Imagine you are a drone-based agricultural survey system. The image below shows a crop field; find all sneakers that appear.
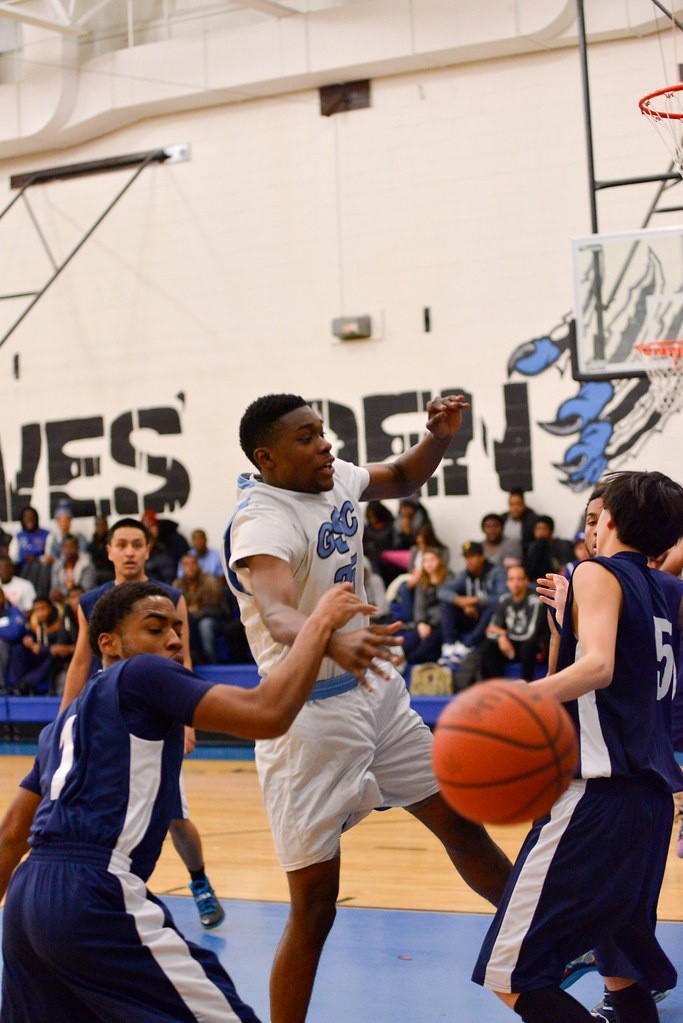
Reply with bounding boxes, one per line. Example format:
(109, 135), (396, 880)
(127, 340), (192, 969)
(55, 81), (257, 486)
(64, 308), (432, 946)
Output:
(189, 876), (225, 930)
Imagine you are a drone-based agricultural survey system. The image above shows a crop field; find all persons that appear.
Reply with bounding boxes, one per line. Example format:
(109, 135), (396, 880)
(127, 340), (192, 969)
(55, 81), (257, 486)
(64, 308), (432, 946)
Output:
(0, 393), (683, 1023)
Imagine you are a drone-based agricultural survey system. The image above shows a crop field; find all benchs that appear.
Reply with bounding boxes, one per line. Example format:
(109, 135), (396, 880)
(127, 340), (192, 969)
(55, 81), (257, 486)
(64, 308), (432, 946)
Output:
(0, 665), (547, 741)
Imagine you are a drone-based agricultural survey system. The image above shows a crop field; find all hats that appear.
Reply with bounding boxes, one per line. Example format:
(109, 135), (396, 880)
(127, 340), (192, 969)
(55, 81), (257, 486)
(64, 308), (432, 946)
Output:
(460, 542), (483, 554)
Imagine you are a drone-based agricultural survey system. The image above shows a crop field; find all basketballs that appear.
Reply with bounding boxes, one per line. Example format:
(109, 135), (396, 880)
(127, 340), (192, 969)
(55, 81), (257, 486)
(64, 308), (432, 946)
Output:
(433, 675), (580, 828)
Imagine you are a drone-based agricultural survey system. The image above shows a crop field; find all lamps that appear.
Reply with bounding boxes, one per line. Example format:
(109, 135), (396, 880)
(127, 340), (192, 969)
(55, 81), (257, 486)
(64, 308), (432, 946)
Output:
(332, 315), (372, 341)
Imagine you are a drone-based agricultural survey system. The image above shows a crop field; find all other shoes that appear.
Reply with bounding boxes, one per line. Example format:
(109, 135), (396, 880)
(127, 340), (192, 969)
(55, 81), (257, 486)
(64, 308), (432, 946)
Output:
(591, 987), (671, 1018)
(12, 681), (29, 696)
(449, 640), (474, 664)
(675, 811), (683, 858)
(559, 949), (600, 989)
(437, 643), (453, 666)
(0, 687), (12, 695)
